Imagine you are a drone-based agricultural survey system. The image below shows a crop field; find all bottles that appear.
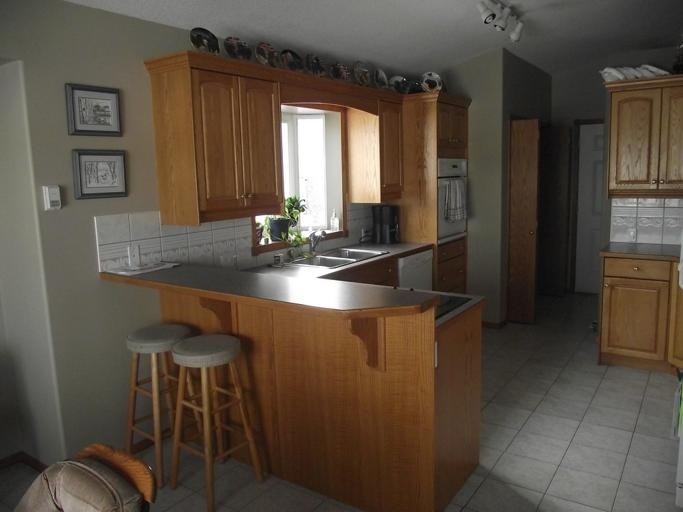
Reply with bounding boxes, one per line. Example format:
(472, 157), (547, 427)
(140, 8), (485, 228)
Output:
(273, 250), (283, 265)
(331, 209), (339, 231)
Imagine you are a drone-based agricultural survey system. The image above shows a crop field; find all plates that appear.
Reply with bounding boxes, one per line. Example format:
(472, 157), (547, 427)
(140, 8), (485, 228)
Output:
(188, 27), (443, 94)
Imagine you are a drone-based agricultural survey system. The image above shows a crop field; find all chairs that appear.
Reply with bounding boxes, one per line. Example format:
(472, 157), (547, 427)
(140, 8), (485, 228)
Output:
(71, 443), (156, 502)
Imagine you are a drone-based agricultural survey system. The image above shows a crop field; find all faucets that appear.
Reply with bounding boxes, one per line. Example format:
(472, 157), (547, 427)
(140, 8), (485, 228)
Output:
(309, 230), (326, 251)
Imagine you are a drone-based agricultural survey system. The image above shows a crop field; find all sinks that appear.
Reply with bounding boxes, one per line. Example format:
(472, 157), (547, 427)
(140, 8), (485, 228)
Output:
(316, 247), (388, 261)
(289, 255), (358, 270)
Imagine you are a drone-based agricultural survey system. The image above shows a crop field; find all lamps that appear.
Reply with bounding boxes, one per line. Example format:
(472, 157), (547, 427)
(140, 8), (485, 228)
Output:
(476, 0), (525, 42)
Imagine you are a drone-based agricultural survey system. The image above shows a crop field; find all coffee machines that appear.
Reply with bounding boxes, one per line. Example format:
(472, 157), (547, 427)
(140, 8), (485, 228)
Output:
(371, 205), (400, 245)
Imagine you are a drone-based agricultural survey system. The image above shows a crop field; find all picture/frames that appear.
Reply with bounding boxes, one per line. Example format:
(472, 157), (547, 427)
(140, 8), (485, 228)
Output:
(65, 83), (122, 137)
(72, 149), (127, 199)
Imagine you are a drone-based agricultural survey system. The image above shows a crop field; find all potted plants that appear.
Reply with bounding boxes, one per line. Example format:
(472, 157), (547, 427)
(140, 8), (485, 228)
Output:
(267, 195), (306, 247)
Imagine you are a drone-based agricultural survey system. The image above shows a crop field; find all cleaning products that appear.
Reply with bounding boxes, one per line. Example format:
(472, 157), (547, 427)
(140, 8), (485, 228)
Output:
(375, 222), (381, 243)
(272, 249), (284, 268)
(330, 208), (339, 232)
(383, 222), (390, 244)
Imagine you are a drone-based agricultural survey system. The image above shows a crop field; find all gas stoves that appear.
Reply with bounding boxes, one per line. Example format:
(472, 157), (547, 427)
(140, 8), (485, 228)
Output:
(389, 286), (472, 320)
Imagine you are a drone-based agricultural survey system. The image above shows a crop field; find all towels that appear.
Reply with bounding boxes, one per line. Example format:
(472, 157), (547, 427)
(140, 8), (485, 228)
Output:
(444, 179), (468, 224)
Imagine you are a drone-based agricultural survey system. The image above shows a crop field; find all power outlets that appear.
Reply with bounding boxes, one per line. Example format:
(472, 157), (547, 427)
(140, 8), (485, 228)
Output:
(362, 228), (368, 243)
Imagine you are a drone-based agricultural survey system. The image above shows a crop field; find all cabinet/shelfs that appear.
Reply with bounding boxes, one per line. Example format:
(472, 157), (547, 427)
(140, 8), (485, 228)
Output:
(664, 255), (683, 375)
(603, 75), (683, 196)
(337, 255), (398, 287)
(346, 84), (402, 204)
(402, 93), (471, 294)
(144, 50), (283, 225)
(597, 251), (668, 374)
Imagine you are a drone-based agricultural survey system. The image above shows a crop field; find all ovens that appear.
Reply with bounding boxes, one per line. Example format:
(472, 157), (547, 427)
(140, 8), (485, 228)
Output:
(438, 156), (468, 245)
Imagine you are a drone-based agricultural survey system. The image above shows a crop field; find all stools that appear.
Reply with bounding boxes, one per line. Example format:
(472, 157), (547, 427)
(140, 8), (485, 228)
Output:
(126, 325), (203, 489)
(171, 334), (262, 511)
